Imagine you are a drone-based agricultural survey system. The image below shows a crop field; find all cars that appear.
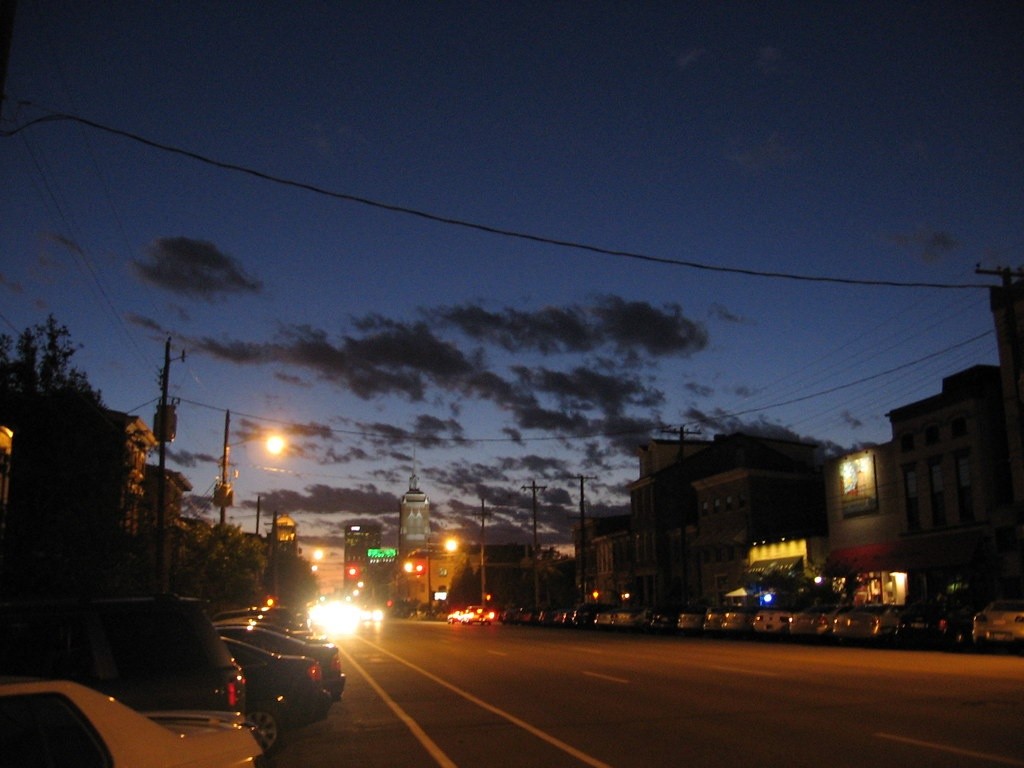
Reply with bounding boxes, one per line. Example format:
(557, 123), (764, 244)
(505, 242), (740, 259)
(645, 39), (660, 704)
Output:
(971, 600), (1024, 656)
(498, 607), (675, 630)
(0, 680), (263, 768)
(447, 610), (465, 623)
(215, 626), (345, 702)
(721, 611), (754, 637)
(873, 600), (953, 650)
(461, 606), (493, 625)
(788, 605), (854, 644)
(833, 603), (903, 646)
(221, 636), (331, 756)
(0, 592), (248, 714)
(752, 608), (793, 639)
(677, 612), (705, 631)
(702, 608), (724, 631)
(211, 606), (327, 644)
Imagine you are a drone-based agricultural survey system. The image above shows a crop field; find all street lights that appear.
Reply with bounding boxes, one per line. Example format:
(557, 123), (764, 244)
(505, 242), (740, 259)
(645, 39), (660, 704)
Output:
(220, 436), (281, 524)
(427, 538), (456, 621)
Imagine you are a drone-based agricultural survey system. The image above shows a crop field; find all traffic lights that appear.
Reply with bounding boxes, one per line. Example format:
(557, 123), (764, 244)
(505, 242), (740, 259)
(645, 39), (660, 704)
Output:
(416, 565), (423, 572)
(405, 563), (412, 572)
(350, 568), (355, 575)
